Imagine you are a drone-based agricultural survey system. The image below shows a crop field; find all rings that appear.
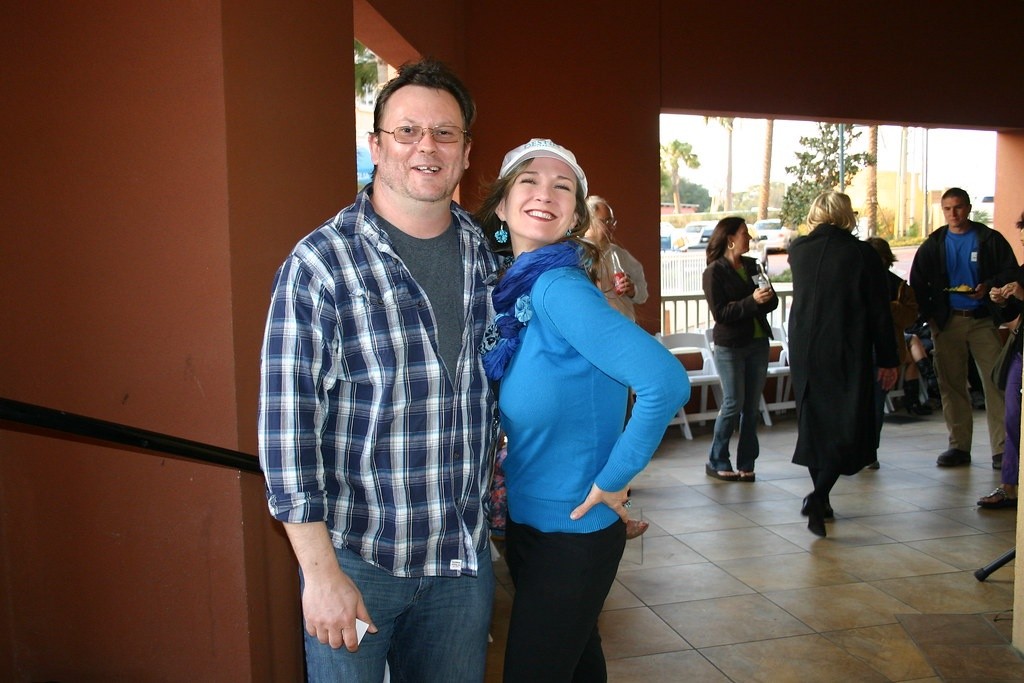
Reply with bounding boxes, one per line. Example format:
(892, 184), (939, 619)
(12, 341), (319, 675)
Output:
(992, 295), (994, 297)
(623, 500), (631, 507)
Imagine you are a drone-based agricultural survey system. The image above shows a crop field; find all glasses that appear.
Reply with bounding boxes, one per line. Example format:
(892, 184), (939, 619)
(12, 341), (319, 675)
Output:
(847, 211), (858, 219)
(377, 126), (469, 144)
(595, 216), (619, 228)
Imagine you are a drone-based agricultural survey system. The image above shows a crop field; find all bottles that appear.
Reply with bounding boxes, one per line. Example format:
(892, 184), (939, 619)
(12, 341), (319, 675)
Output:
(756, 261), (770, 289)
(610, 251), (625, 295)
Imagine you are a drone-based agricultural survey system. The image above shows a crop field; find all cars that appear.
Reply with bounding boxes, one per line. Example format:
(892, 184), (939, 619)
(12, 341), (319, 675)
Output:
(658, 220), (721, 249)
(753, 219), (792, 254)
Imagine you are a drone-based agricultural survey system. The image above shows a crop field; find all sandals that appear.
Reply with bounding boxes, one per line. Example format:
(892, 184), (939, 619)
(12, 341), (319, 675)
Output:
(626, 517), (648, 539)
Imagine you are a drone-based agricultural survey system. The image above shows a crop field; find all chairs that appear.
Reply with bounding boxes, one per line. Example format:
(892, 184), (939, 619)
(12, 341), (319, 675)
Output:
(631, 319), (935, 441)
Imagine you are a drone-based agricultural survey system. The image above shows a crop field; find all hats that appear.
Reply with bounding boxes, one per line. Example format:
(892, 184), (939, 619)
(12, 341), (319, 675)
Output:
(499, 137), (589, 201)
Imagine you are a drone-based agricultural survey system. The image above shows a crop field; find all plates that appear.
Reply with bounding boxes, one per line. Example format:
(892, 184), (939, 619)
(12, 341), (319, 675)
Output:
(942, 290), (978, 294)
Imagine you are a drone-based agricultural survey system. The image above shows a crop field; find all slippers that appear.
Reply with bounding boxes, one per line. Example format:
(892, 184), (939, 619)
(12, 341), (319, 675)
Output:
(976, 482), (1015, 509)
(734, 466), (755, 482)
(705, 462), (735, 482)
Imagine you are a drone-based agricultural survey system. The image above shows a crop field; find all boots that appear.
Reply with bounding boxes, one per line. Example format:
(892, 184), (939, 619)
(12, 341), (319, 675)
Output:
(916, 355), (938, 378)
(903, 378), (932, 416)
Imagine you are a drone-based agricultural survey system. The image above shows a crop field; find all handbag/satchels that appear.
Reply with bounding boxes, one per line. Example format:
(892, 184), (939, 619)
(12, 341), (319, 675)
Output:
(990, 330), (1020, 391)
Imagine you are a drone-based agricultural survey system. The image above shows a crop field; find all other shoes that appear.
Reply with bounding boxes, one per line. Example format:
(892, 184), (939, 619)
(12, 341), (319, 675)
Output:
(992, 453), (1004, 470)
(939, 447), (972, 465)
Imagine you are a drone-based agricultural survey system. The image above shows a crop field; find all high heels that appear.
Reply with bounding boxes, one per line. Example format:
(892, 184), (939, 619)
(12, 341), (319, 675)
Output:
(801, 490), (833, 538)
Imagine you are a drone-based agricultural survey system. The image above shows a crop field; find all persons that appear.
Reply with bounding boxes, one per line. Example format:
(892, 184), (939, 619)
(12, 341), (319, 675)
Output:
(787, 192), (901, 537)
(901, 333), (933, 415)
(473, 138), (691, 683)
(910, 188), (1019, 467)
(865, 237), (918, 469)
(702, 217), (779, 482)
(254, 63), (501, 683)
(580, 196), (648, 322)
(977, 213), (1024, 508)
